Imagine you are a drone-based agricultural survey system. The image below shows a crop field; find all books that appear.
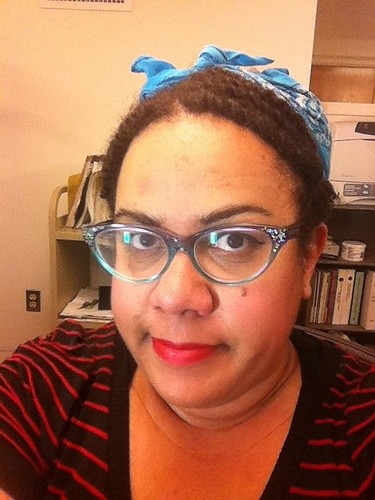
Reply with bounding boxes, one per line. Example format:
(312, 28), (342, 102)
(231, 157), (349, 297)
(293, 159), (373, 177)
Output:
(305, 263), (375, 327)
(64, 153), (111, 229)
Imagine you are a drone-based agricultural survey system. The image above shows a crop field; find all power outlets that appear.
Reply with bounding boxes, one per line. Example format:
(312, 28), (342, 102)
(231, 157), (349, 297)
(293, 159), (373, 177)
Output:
(25, 289), (41, 312)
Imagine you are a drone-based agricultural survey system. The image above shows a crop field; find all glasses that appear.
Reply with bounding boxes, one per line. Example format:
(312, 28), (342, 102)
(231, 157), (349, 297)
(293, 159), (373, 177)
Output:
(82, 220), (306, 287)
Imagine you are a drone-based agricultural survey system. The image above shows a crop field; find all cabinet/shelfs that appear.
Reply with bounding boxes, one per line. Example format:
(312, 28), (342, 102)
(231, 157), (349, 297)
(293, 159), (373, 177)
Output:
(48, 185), (375, 351)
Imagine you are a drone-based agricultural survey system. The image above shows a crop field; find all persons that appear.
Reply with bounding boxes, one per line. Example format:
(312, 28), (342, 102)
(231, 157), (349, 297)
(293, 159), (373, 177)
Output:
(0, 47), (373, 499)
